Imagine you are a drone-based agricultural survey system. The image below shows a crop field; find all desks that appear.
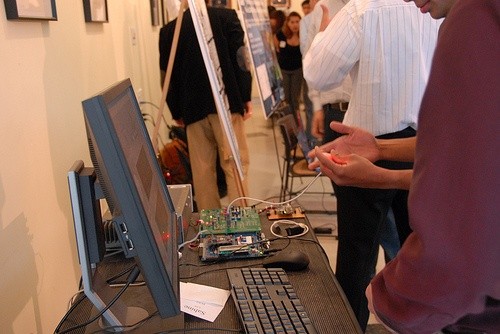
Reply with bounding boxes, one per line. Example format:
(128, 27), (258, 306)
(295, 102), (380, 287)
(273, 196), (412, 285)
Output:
(57, 212), (362, 334)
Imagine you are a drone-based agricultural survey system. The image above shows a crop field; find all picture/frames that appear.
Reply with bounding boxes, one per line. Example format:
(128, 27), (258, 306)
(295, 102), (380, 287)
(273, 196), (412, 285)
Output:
(3, 0), (58, 21)
(82, 0), (109, 23)
(150, 0), (168, 26)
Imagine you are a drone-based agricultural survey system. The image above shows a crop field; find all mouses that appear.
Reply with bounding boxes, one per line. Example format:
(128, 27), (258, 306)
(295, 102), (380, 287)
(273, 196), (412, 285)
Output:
(263, 250), (310, 270)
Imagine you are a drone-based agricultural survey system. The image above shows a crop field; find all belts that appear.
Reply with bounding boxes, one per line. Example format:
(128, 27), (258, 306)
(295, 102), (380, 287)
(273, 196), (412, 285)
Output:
(330, 102), (349, 111)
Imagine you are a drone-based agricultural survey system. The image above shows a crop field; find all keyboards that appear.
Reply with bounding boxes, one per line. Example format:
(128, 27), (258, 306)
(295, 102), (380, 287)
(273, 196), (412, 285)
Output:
(226, 267), (318, 334)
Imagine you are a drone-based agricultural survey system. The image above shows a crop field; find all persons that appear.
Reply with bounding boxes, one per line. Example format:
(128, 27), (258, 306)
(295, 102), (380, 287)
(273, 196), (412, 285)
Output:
(269, 0), (349, 143)
(364, 0), (500, 334)
(307, 121), (417, 190)
(303, 0), (445, 334)
(159, 0), (253, 214)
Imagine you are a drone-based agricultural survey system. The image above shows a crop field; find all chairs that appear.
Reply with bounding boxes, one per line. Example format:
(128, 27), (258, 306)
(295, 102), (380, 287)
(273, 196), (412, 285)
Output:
(276, 114), (337, 215)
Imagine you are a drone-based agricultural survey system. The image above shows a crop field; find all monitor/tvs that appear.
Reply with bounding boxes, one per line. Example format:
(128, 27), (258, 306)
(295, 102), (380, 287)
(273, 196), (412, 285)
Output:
(68, 78), (181, 334)
